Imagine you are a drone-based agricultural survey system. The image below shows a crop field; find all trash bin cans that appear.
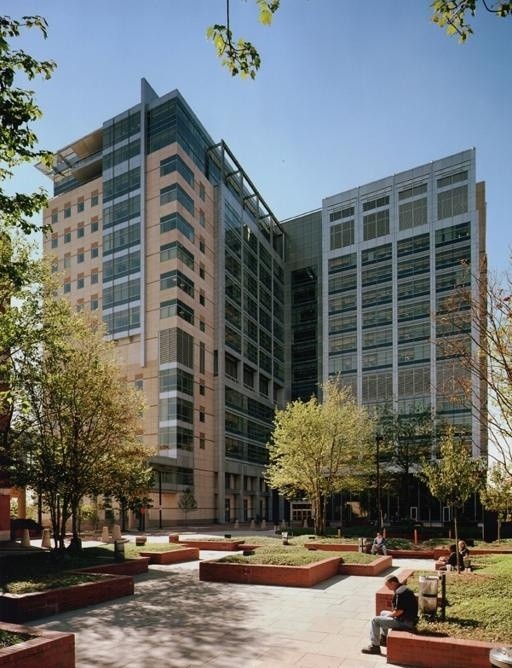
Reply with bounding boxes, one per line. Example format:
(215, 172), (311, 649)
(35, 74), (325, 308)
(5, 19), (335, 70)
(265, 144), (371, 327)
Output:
(282, 532), (289, 545)
(114, 541), (124, 561)
(489, 646), (512, 668)
(358, 538), (371, 554)
(418, 575), (438, 621)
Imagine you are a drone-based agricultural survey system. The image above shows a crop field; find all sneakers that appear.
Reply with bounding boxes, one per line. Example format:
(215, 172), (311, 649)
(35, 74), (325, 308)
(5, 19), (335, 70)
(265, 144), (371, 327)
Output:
(379, 634), (386, 646)
(362, 643), (380, 654)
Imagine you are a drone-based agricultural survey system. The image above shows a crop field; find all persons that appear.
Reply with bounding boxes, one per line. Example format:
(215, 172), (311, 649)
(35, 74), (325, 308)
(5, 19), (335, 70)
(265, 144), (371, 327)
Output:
(438, 543), (466, 572)
(370, 530), (389, 555)
(456, 541), (470, 567)
(362, 575), (419, 654)
(365, 538), (373, 554)
